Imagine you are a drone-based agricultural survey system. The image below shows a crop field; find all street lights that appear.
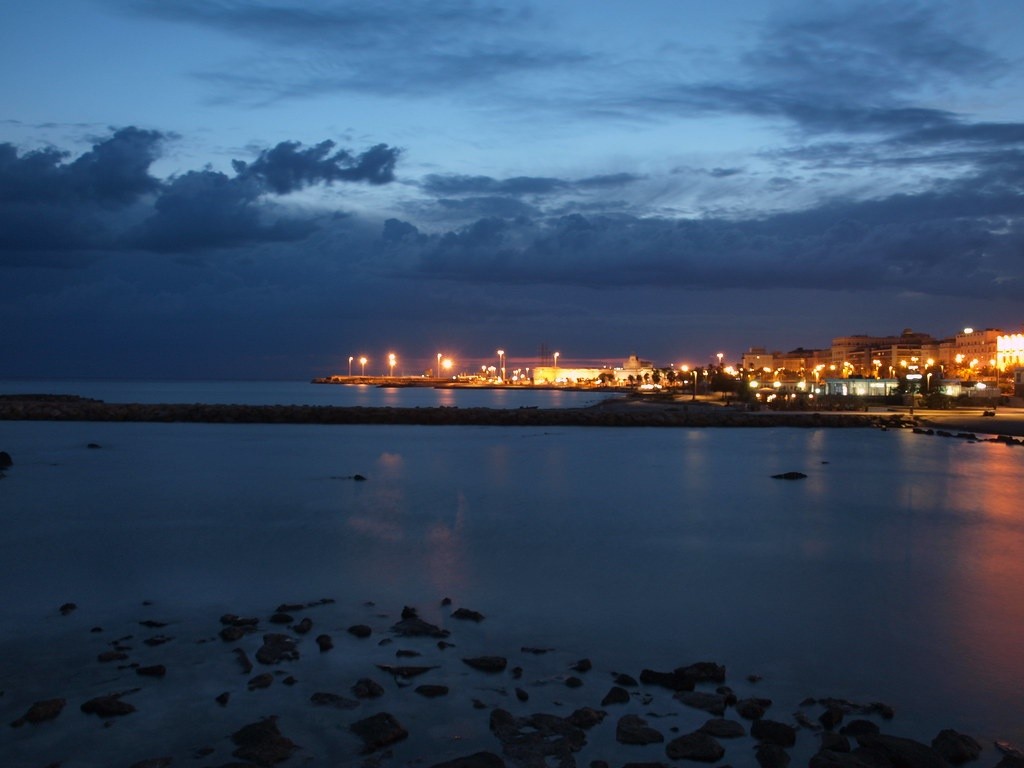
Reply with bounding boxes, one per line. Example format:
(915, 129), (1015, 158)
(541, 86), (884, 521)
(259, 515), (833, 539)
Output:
(437, 353), (442, 379)
(926, 373), (933, 392)
(717, 353), (723, 366)
(361, 358), (367, 376)
(553, 352), (559, 383)
(692, 371), (697, 402)
(349, 356), (353, 377)
(389, 354), (394, 376)
(497, 350), (504, 379)
